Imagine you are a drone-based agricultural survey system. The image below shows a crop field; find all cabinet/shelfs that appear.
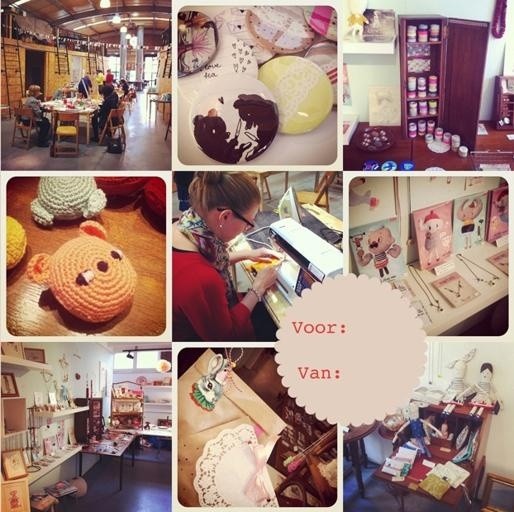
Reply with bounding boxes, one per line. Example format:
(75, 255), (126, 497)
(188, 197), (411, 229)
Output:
(398, 16), (491, 139)
(144, 386), (173, 431)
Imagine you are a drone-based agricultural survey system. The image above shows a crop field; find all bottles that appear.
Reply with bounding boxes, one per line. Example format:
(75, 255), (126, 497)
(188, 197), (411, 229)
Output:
(406, 21), (469, 159)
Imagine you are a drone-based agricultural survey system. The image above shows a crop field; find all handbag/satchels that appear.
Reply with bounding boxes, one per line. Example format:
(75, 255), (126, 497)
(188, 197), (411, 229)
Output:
(106, 135), (122, 153)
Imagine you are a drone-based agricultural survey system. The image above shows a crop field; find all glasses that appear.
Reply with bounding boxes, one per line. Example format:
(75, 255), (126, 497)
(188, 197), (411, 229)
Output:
(217, 208), (254, 231)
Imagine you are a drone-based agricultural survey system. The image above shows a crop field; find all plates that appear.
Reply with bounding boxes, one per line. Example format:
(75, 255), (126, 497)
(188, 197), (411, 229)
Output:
(178, 6), (337, 164)
(354, 126), (394, 152)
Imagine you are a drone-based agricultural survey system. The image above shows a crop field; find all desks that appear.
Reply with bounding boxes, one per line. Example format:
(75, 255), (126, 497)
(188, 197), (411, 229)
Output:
(224, 202), (342, 329)
(78, 423), (172, 490)
(374, 445), (474, 512)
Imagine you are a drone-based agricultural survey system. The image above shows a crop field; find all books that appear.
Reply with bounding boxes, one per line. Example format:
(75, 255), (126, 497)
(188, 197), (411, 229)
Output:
(348, 184), (508, 312)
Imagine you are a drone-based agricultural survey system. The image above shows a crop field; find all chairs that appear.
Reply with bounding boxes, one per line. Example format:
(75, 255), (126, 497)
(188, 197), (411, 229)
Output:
(294, 171), (336, 213)
(10, 78), (135, 158)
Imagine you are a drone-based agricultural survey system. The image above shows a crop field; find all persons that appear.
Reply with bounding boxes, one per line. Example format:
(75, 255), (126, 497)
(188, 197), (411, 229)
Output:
(89, 85), (118, 143)
(20, 84), (50, 147)
(172, 169), (282, 342)
(50, 69), (133, 100)
(460, 361), (503, 422)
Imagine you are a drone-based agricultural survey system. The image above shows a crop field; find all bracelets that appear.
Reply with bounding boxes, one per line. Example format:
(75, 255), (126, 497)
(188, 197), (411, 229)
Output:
(246, 287), (261, 303)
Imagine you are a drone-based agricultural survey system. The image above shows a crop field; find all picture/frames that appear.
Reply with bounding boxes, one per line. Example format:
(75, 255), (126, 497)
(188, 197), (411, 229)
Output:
(0, 448), (32, 512)
(1, 373), (20, 397)
(24, 348), (44, 364)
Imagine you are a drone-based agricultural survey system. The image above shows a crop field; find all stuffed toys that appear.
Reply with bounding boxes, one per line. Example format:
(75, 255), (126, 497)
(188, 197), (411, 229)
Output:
(391, 401), (443, 459)
(438, 360), (470, 419)
